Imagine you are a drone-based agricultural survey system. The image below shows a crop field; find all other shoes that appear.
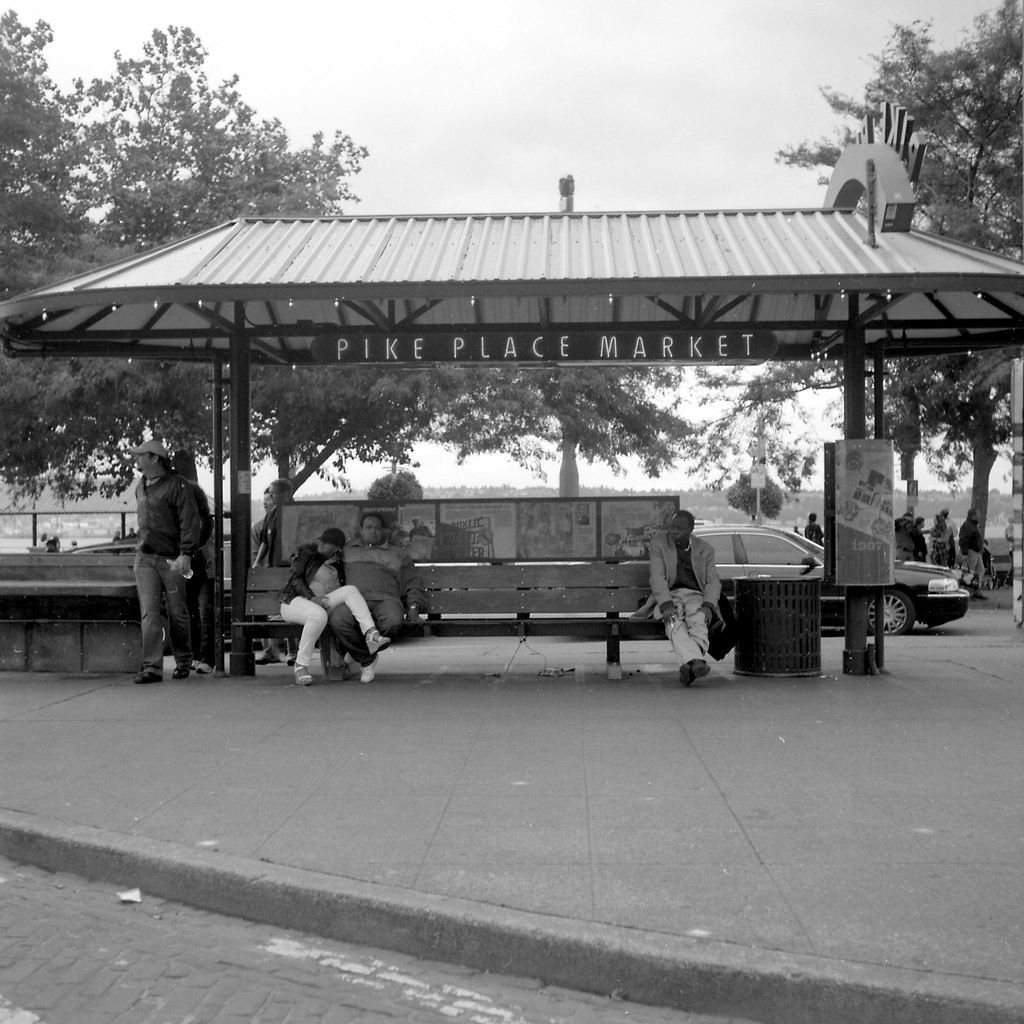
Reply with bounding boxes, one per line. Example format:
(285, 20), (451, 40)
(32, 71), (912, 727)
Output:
(288, 657), (297, 665)
(678, 662), (709, 687)
(252, 656), (282, 665)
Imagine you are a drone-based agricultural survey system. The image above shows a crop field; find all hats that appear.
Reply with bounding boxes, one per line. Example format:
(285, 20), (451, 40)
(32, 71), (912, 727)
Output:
(130, 441), (166, 457)
(317, 527), (345, 546)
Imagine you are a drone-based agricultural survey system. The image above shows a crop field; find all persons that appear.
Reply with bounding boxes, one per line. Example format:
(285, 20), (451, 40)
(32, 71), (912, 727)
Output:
(47, 539), (59, 553)
(285, 513), (426, 682)
(896, 507), (957, 569)
(959, 506), (988, 600)
(166, 466), (215, 673)
(113, 530), (121, 542)
(251, 486), (281, 665)
(131, 441), (201, 686)
(408, 518), (432, 541)
(252, 478), (300, 665)
(805, 513), (823, 545)
(629, 511), (723, 687)
(279, 526), (390, 686)
(128, 527), (137, 537)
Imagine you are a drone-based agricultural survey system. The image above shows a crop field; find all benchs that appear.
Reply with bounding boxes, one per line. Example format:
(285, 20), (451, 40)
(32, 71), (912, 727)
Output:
(232, 560), (668, 682)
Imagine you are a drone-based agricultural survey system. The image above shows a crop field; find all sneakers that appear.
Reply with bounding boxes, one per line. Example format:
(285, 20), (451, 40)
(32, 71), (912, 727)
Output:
(190, 660), (215, 674)
(362, 655), (379, 682)
(345, 651), (357, 663)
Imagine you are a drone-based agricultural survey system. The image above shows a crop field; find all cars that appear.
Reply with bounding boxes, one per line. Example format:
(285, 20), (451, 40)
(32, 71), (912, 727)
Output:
(692, 524), (971, 636)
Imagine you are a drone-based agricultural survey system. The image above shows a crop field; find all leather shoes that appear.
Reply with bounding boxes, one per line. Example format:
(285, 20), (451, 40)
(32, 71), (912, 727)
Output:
(172, 668), (190, 678)
(135, 671), (164, 683)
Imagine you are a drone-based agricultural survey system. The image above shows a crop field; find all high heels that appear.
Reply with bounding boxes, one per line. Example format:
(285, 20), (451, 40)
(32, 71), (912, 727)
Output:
(294, 663), (312, 686)
(366, 631), (390, 653)
